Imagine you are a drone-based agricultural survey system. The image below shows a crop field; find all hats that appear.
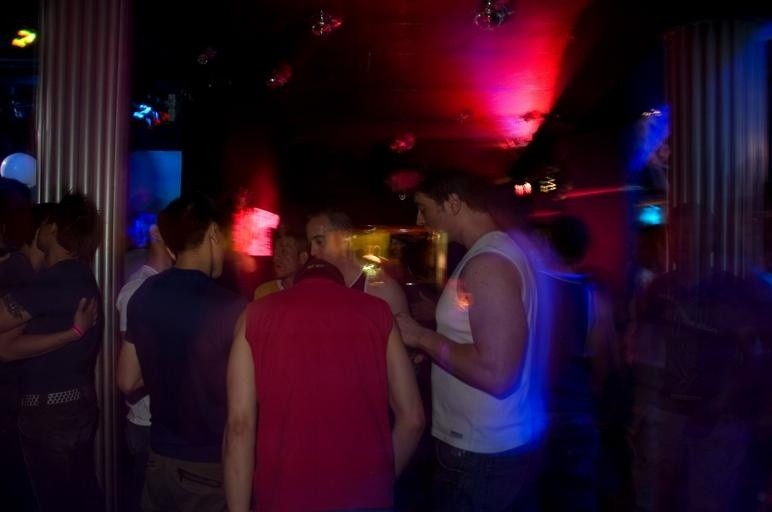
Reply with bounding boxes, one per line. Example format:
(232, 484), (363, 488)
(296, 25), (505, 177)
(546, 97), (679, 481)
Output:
(276, 219), (309, 241)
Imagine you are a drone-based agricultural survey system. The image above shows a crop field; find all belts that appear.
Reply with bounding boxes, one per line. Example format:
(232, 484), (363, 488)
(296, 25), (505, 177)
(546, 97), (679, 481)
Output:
(17, 384), (88, 408)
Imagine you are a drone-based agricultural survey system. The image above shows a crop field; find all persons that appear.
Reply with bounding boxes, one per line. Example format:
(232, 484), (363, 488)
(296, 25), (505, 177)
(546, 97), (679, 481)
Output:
(4, 163), (770, 512)
